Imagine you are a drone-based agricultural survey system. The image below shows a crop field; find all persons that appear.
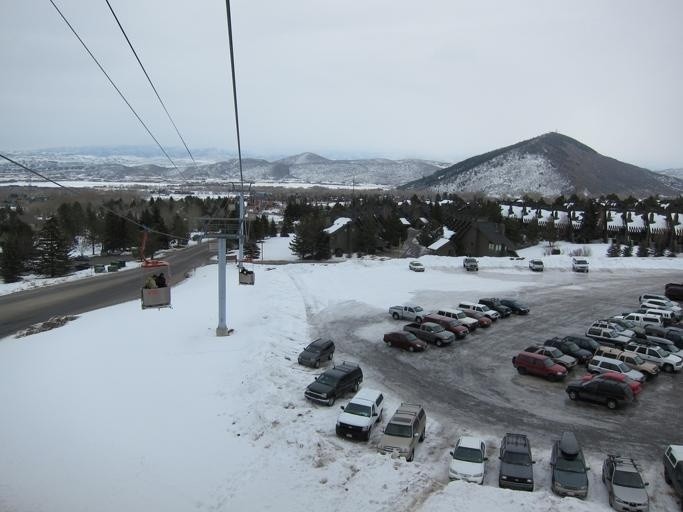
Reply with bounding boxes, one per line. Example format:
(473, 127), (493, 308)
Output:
(155, 272), (167, 287)
(145, 275), (157, 288)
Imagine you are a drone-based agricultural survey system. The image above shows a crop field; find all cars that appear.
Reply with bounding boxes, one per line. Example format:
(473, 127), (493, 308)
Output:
(409, 261), (425, 272)
(529, 259), (544, 271)
(463, 257), (479, 271)
(572, 257), (589, 272)
